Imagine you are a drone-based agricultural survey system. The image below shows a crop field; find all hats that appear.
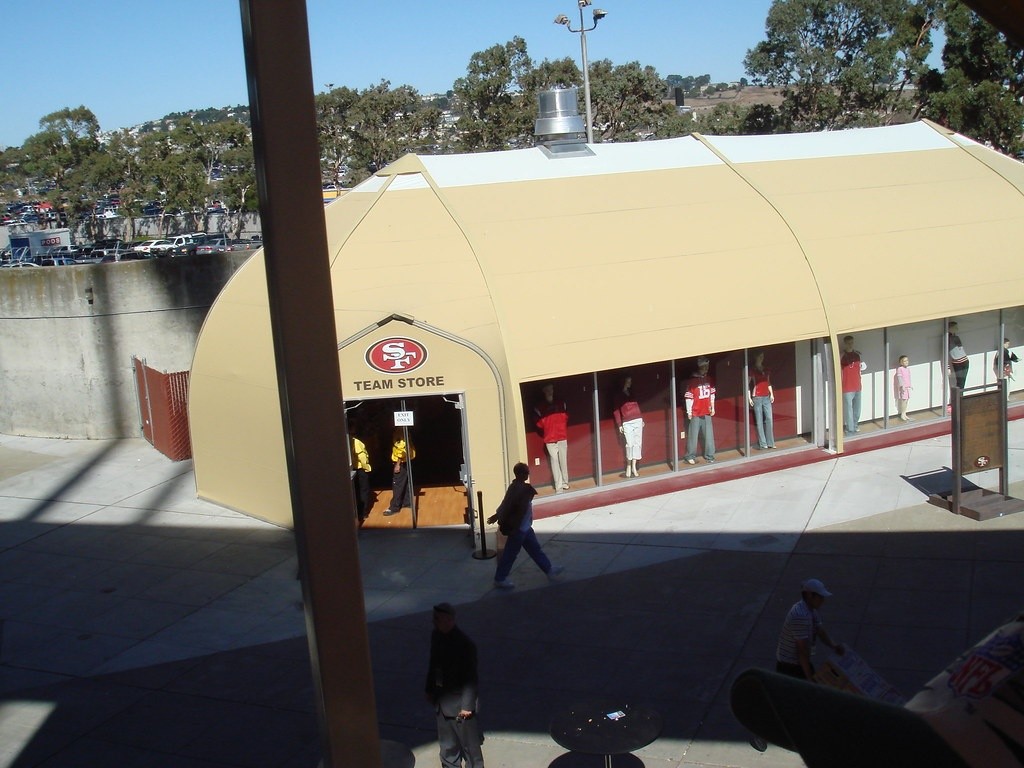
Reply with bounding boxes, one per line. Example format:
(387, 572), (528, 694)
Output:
(698, 357), (709, 366)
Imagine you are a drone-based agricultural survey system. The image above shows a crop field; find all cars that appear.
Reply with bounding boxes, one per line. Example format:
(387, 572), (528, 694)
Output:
(321, 159), (353, 189)
(0, 191), (262, 267)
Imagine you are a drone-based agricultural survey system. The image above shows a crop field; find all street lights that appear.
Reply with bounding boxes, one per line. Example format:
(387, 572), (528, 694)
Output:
(555, 1), (608, 144)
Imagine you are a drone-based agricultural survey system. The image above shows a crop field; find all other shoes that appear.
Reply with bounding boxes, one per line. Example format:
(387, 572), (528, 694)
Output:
(358, 513), (368, 523)
(495, 579), (515, 588)
(383, 509), (397, 515)
(400, 504), (408, 507)
(548, 566), (564, 577)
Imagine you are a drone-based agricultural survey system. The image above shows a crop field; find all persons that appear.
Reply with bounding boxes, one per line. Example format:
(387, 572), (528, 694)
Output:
(493, 463), (564, 587)
(383, 419), (417, 516)
(754, 580), (843, 749)
(425, 602), (483, 768)
(896, 356), (910, 420)
(994, 338), (1022, 402)
(948, 322), (969, 388)
(685, 358), (716, 463)
(841, 336), (867, 432)
(748, 351), (776, 449)
(535, 383), (569, 494)
(614, 374), (645, 477)
(349, 418), (371, 517)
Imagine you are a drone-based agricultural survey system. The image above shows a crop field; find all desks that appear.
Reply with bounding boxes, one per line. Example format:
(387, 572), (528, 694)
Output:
(550, 699), (663, 768)
